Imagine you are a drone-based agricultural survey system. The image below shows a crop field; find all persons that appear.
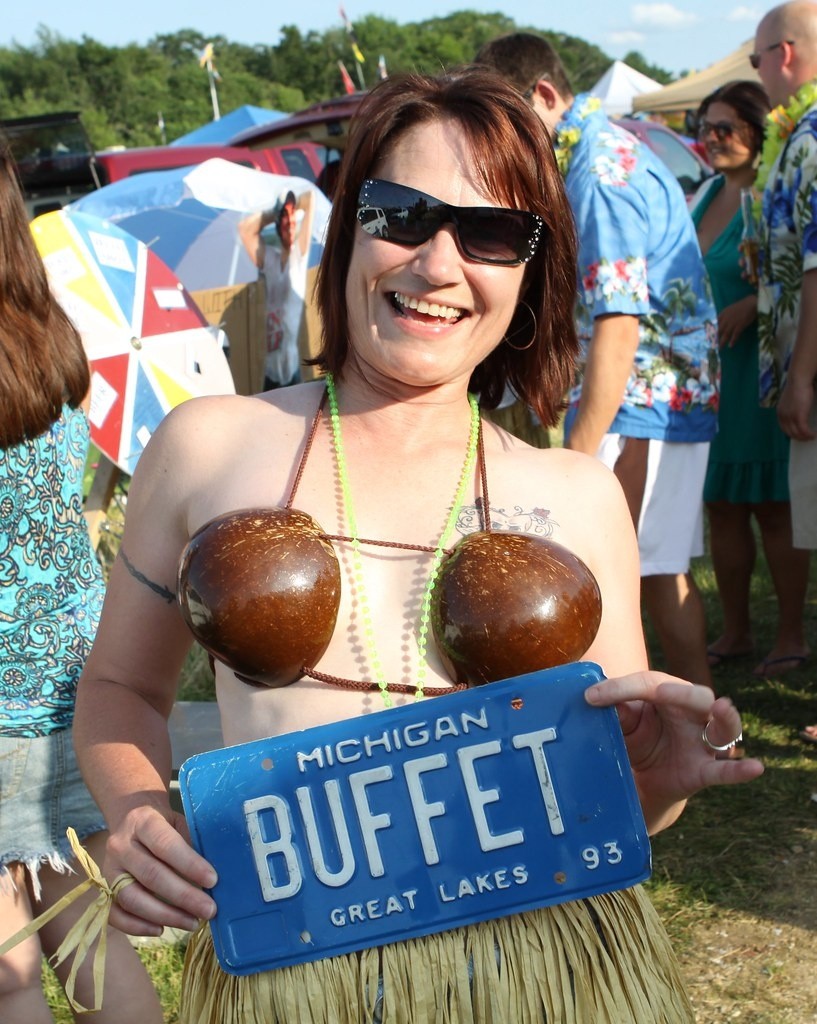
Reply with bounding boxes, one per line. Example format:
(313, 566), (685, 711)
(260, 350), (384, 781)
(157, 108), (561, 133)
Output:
(1, 146), (167, 1022)
(461, 2), (817, 739)
(70, 66), (770, 1023)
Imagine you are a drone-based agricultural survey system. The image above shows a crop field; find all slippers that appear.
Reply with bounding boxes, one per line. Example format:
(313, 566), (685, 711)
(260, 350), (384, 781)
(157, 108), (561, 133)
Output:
(758, 650), (811, 677)
(706, 649), (760, 670)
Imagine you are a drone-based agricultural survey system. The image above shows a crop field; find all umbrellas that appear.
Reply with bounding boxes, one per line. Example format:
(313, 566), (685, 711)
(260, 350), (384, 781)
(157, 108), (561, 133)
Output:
(65, 158), (336, 289)
(28, 205), (238, 479)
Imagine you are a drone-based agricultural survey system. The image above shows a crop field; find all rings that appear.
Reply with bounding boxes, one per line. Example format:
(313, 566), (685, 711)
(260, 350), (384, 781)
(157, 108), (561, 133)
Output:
(702, 717), (743, 752)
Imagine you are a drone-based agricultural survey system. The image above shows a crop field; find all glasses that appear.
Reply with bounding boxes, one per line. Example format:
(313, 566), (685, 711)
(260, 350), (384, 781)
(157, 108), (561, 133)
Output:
(750, 40), (794, 70)
(697, 119), (750, 142)
(354, 177), (543, 267)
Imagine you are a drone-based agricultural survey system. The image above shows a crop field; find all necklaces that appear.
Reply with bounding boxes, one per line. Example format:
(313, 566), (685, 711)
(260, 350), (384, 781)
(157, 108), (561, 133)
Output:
(324, 369), (485, 707)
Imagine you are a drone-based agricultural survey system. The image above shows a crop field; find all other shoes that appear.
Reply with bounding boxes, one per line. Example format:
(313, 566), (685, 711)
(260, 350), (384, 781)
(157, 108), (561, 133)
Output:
(799, 726), (817, 741)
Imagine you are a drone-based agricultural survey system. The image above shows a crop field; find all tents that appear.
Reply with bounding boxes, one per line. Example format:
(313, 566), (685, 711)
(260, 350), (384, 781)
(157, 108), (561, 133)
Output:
(629, 33), (762, 116)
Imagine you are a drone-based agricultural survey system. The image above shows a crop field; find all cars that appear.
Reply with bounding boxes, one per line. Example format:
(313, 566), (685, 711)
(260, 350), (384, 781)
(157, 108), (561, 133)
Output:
(391, 207), (409, 221)
(616, 118), (714, 206)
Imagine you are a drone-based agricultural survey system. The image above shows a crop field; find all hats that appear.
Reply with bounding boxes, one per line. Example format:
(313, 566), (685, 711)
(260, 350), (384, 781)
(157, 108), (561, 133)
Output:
(275, 189), (297, 231)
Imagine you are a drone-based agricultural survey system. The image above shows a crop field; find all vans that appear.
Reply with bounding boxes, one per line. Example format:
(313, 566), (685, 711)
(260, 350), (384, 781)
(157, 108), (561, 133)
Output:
(356, 207), (389, 238)
